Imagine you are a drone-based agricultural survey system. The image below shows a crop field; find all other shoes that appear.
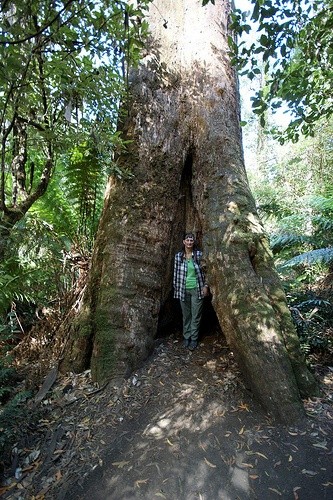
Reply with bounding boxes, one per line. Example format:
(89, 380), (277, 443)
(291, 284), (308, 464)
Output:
(189, 340), (198, 350)
(182, 338), (189, 349)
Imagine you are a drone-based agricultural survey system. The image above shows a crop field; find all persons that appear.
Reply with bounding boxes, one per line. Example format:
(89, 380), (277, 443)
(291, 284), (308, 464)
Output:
(172, 234), (210, 351)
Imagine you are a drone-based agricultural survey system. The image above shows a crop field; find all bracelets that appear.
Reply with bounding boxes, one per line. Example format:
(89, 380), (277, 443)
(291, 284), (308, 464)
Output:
(204, 285), (209, 288)
